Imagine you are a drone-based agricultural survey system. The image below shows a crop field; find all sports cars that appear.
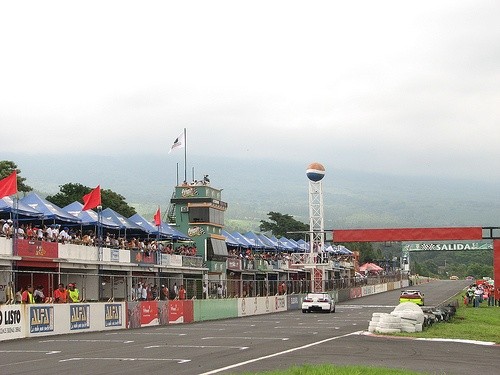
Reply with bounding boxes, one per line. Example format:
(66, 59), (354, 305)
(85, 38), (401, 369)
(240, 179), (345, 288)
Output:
(301, 292), (335, 313)
(399, 290), (424, 306)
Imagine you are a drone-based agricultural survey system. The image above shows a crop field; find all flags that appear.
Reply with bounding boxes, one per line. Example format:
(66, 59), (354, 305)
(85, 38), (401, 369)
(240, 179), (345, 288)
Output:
(0, 170), (17, 199)
(168, 131), (184, 154)
(154, 208), (161, 226)
(82, 185), (101, 211)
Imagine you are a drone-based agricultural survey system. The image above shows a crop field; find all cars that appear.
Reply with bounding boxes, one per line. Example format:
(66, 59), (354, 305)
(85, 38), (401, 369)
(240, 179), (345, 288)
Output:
(466, 277), (473, 280)
(450, 276), (459, 280)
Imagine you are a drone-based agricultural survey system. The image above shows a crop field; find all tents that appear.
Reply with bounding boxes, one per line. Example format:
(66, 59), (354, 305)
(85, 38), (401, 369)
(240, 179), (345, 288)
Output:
(359, 262), (384, 272)
(0, 192), (353, 254)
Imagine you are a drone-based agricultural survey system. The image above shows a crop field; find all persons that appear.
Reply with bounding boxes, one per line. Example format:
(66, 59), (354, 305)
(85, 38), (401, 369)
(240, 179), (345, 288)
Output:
(73, 283), (77, 289)
(21, 285), (35, 304)
(16, 287), (23, 304)
(131, 281), (186, 301)
(68, 282), (82, 303)
(54, 283), (69, 303)
(182, 175), (211, 187)
(33, 284), (45, 303)
(203, 249), (412, 299)
(0, 219), (196, 256)
(4, 281), (15, 305)
(465, 281), (500, 307)
